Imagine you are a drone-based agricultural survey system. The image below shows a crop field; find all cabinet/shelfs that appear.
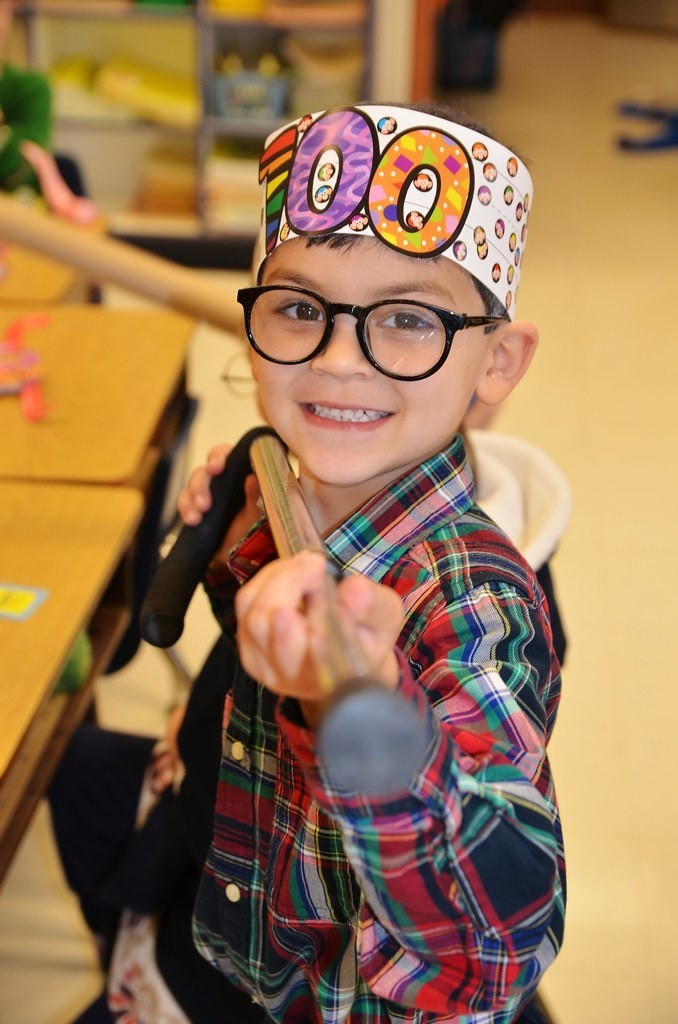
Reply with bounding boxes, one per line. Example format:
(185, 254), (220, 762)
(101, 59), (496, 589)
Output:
(0, 0), (442, 261)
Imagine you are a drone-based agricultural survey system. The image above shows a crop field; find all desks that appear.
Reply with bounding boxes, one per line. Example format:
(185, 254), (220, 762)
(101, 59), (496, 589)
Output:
(1, 481), (147, 891)
(0, 301), (194, 486)
(0, 192), (108, 299)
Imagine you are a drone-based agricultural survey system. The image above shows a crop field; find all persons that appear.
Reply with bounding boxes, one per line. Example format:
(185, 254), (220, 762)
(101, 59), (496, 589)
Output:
(44, 100), (568, 1024)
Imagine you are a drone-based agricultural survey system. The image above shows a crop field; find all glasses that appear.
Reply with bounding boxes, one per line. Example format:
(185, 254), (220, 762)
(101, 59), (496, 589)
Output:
(238, 287), (509, 381)
